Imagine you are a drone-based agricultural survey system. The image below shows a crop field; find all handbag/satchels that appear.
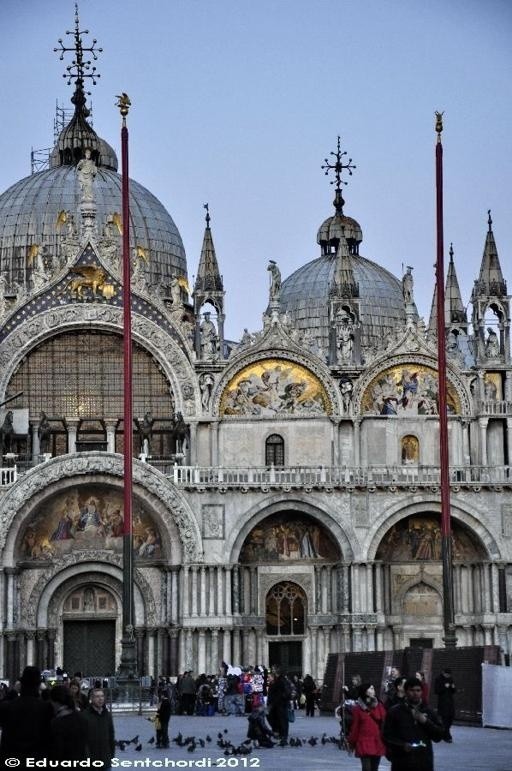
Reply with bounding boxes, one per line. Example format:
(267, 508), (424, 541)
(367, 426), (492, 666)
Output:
(288, 704), (295, 722)
(155, 718), (161, 730)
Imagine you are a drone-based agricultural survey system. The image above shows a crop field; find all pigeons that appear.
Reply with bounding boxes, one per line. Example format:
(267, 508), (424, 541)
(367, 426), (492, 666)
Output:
(115, 734), (142, 752)
(279, 732), (341, 748)
(172, 728), (253, 757)
(148, 736), (154, 745)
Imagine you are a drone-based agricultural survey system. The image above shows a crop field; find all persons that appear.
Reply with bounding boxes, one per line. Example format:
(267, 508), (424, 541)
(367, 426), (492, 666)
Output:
(127, 668), (330, 717)
(1, 216), (192, 357)
(342, 672), (363, 701)
(74, 146), (98, 203)
(384, 676), (446, 771)
(401, 267), (414, 302)
(383, 676), (408, 711)
(431, 666), (457, 746)
(246, 702), (280, 748)
(346, 682), (388, 771)
(156, 689), (171, 748)
(383, 666), (402, 694)
(412, 670), (430, 705)
(193, 307), (512, 417)
(245, 514), (477, 562)
(267, 261), (282, 296)
(266, 663), (292, 747)
(0, 658), (117, 771)
(143, 709), (162, 745)
(23, 490), (162, 557)
